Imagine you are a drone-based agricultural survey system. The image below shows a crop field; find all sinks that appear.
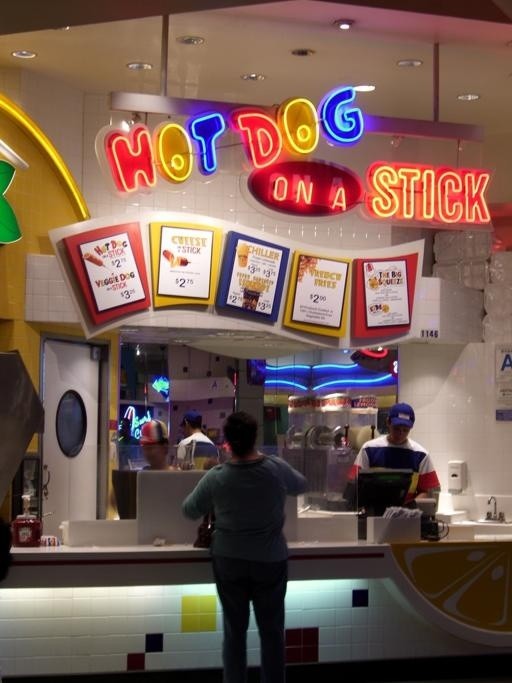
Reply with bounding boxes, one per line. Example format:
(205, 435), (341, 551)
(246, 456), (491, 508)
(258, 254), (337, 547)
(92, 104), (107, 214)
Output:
(436, 521), (512, 540)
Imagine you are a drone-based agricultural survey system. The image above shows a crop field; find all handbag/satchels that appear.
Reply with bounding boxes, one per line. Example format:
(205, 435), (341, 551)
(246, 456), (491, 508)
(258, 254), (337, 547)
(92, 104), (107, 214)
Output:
(193, 506), (216, 549)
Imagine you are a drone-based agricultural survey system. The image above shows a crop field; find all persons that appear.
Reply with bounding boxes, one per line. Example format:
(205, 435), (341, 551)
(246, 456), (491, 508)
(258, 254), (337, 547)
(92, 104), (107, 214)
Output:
(140, 420), (179, 469)
(182, 412), (308, 683)
(343, 404), (441, 510)
(176, 411), (219, 466)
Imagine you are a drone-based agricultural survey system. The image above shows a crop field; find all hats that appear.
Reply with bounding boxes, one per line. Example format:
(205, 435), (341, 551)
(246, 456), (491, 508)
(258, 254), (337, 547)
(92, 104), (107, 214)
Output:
(388, 402), (415, 426)
(179, 411), (202, 428)
(138, 419), (170, 446)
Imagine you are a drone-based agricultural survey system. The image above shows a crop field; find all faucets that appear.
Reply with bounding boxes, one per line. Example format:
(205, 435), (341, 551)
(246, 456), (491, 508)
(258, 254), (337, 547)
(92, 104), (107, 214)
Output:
(488, 496), (498, 520)
